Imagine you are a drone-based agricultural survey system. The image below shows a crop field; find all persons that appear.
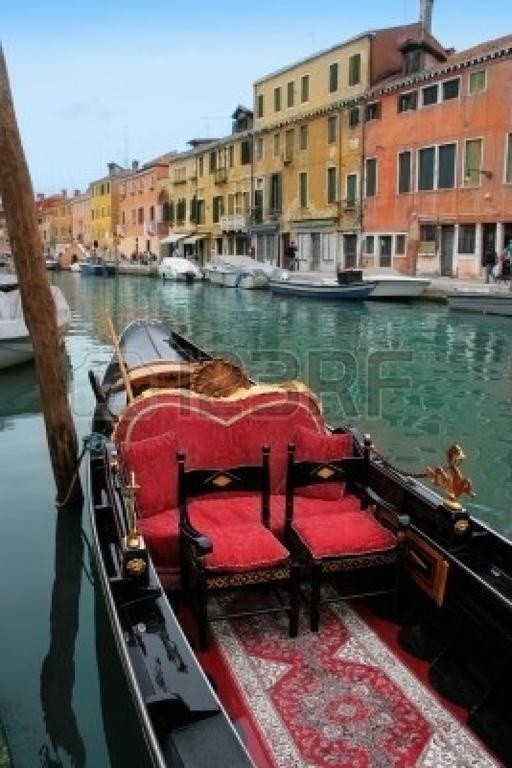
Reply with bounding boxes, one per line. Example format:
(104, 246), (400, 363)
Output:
(482, 246), (497, 284)
(286, 241), (300, 271)
(498, 248), (511, 285)
(128, 249), (161, 267)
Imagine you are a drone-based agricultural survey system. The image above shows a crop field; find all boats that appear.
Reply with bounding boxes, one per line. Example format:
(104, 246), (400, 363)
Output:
(85, 317), (510, 768)
(337, 271), (429, 299)
(205, 256), (280, 288)
(45, 254), (57, 268)
(77, 255), (116, 276)
(1, 278), (69, 368)
(269, 279), (376, 299)
(158, 256), (202, 282)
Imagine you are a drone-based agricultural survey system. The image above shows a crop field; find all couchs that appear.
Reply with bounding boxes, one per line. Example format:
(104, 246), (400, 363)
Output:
(112, 381), (363, 590)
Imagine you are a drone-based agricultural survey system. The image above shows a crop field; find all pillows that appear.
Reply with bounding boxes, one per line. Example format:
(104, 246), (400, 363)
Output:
(276, 424), (353, 504)
(121, 429), (180, 519)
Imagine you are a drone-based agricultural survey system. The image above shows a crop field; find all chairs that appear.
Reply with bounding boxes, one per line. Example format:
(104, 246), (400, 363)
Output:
(175, 433), (414, 653)
